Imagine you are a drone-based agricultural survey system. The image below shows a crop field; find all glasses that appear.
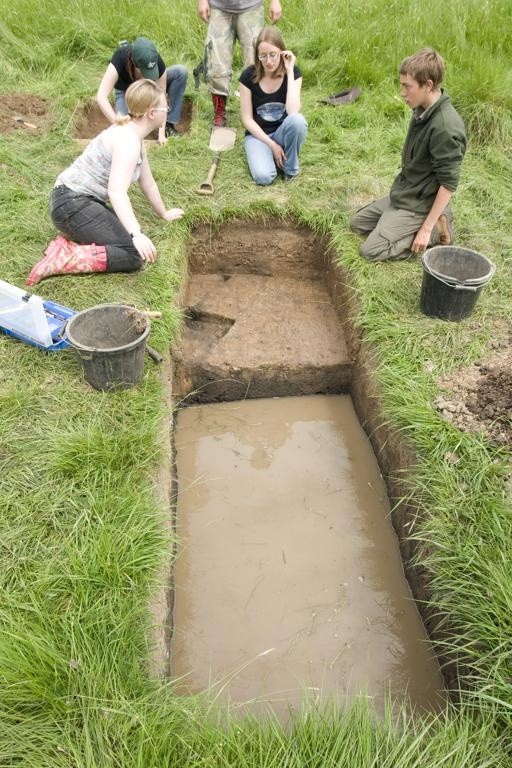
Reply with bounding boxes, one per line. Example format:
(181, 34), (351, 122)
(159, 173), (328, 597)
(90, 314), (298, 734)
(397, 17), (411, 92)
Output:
(144, 106), (171, 117)
(257, 50), (280, 61)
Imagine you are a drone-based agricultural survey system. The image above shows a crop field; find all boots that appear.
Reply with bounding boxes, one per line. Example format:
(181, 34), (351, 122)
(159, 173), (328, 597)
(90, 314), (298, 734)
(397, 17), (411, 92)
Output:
(211, 94), (228, 128)
(25, 234), (107, 288)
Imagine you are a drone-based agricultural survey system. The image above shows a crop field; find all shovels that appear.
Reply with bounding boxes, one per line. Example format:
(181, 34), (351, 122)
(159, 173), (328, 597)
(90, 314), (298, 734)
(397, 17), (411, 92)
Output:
(194, 126), (237, 195)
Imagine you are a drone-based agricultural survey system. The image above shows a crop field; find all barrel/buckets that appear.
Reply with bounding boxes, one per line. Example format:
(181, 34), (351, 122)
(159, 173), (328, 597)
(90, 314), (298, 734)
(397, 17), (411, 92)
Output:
(58, 302), (150, 394)
(419, 243), (496, 322)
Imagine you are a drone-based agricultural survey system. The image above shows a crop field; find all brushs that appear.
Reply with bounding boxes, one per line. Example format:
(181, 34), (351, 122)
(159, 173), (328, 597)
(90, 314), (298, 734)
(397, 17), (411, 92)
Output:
(17, 119), (37, 129)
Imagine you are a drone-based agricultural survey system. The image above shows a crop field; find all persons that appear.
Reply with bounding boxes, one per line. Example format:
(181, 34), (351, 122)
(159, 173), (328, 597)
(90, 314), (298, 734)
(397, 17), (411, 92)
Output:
(195, 0), (283, 128)
(237, 26), (309, 186)
(349, 47), (468, 263)
(26, 78), (185, 284)
(95, 36), (188, 147)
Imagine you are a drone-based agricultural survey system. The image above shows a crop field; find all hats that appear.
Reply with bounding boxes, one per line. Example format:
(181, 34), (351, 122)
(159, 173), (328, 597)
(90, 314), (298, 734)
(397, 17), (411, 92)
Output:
(132, 37), (160, 80)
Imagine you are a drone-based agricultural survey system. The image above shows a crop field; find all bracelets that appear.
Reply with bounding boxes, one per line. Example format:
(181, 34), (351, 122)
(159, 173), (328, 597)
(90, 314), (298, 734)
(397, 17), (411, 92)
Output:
(130, 229), (143, 239)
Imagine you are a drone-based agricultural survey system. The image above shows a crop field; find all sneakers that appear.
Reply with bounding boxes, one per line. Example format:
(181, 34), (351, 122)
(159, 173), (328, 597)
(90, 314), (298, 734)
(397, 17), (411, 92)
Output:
(165, 121), (179, 139)
(282, 170), (294, 182)
(436, 208), (454, 248)
